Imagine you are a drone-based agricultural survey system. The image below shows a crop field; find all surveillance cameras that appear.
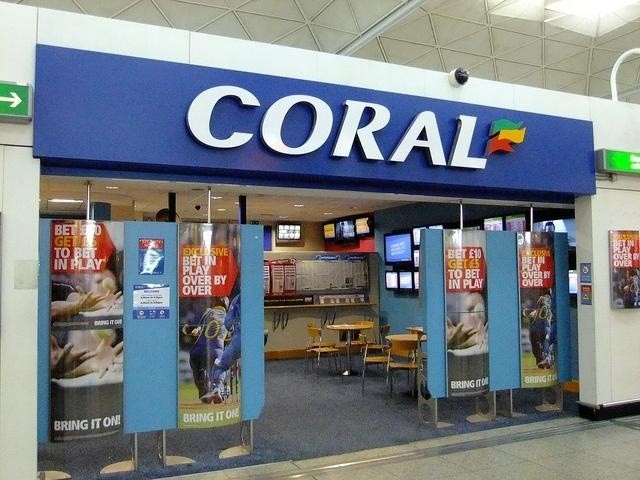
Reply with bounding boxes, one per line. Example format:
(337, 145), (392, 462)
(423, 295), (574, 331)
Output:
(448, 64), (470, 89)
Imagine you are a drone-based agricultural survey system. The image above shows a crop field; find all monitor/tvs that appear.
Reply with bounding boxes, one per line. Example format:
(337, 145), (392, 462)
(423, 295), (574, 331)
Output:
(275, 222), (302, 245)
(322, 215), (375, 243)
(399, 270), (413, 289)
(413, 224), (444, 290)
(383, 230), (411, 264)
(386, 270), (398, 290)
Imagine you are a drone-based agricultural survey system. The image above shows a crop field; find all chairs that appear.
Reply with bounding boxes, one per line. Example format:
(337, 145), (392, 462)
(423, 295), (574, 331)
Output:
(300, 317), (428, 399)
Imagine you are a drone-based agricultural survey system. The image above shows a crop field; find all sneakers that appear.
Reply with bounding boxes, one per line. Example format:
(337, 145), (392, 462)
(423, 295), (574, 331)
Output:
(538, 358), (552, 370)
(199, 390), (225, 405)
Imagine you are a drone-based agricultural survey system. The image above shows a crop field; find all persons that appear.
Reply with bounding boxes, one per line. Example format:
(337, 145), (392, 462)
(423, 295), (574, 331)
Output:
(48, 271), (123, 384)
(616, 269), (639, 307)
(448, 292), (487, 353)
(199, 292), (240, 404)
(189, 295), (230, 403)
(522, 294), (551, 367)
(533, 289), (558, 368)
